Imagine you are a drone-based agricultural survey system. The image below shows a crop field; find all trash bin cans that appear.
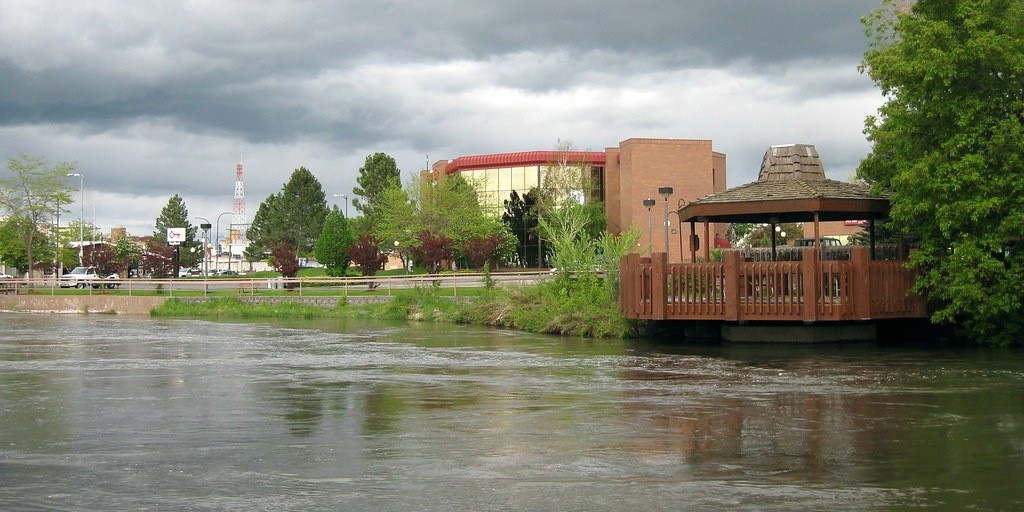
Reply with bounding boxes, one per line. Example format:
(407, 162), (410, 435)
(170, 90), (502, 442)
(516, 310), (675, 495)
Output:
(276, 277), (284, 289)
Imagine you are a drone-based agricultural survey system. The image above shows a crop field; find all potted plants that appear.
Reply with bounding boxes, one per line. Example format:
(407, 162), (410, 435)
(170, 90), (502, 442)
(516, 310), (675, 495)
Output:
(709, 244), (726, 290)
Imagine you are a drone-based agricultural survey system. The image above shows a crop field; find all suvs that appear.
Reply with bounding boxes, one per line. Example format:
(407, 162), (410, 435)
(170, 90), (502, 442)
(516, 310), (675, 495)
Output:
(57, 266), (121, 289)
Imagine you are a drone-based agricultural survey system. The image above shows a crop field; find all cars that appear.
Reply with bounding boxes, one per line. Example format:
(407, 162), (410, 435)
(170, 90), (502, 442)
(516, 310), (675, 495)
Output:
(177, 267), (239, 278)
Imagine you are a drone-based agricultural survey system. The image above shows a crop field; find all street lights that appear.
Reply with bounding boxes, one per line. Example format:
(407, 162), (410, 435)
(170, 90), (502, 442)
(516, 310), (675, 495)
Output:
(332, 194), (348, 219)
(67, 173), (83, 267)
(200, 224), (212, 293)
(194, 217), (212, 273)
(215, 211), (235, 273)
(49, 195), (58, 286)
(658, 187), (674, 263)
(642, 197), (656, 259)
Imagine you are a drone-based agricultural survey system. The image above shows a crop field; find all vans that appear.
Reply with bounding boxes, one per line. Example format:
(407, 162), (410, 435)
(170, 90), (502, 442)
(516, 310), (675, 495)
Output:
(790, 237), (849, 262)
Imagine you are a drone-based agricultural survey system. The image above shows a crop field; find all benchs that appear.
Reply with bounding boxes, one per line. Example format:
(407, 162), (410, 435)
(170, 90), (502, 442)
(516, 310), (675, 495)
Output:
(237, 282), (260, 294)
